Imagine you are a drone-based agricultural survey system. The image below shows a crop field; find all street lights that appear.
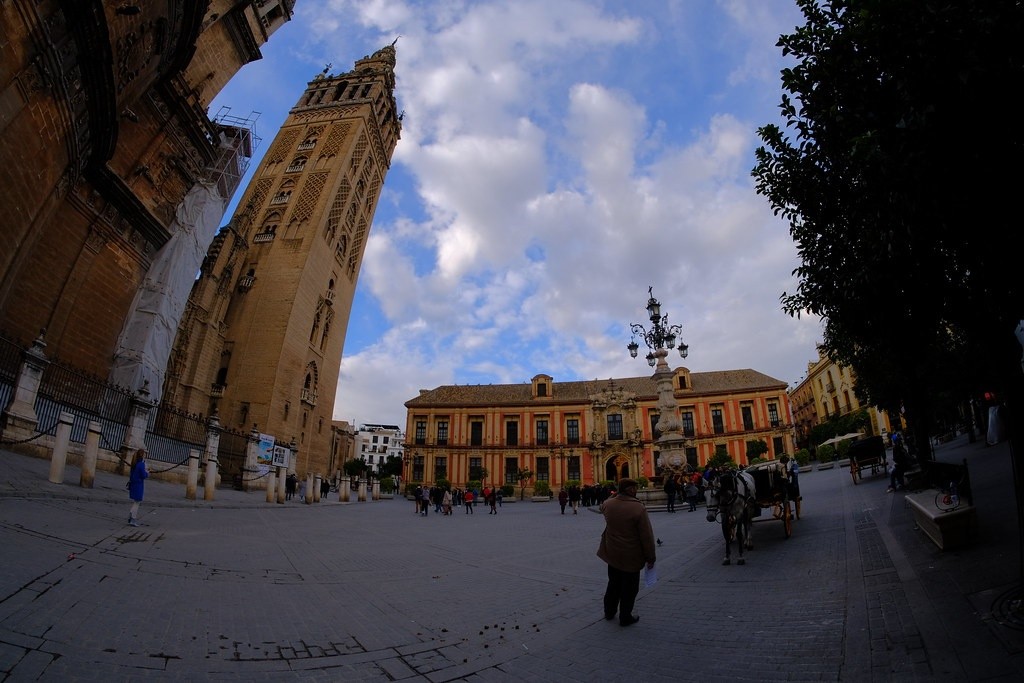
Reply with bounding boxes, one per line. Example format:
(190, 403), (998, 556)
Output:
(626, 284), (693, 487)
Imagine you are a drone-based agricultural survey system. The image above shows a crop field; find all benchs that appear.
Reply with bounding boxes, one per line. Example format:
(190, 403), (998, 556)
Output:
(901, 463), (924, 490)
(904, 458), (983, 552)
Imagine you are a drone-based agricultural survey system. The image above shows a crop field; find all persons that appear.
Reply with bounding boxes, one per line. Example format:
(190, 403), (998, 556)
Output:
(594, 476), (657, 626)
(787, 456), (798, 489)
(126, 448), (150, 527)
(887, 432), (918, 493)
(831, 452), (841, 471)
(286, 465), (729, 516)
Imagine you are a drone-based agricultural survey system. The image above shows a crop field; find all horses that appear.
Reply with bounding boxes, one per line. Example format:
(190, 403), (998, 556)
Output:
(700, 471), (757, 566)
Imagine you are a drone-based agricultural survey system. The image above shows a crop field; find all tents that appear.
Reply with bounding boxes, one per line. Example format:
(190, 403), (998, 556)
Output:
(817, 432), (864, 449)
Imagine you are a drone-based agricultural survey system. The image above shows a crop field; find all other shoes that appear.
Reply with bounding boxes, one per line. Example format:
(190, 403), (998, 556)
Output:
(672, 510), (676, 513)
(620, 615), (639, 627)
(887, 484), (900, 492)
(688, 509), (693, 512)
(694, 508), (696, 511)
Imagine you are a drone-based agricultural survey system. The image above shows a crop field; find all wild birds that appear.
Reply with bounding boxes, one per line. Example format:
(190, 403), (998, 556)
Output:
(657, 537), (663, 546)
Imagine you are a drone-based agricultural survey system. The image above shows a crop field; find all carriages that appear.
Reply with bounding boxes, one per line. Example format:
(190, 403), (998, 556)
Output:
(847, 434), (890, 486)
(701, 451), (803, 565)
